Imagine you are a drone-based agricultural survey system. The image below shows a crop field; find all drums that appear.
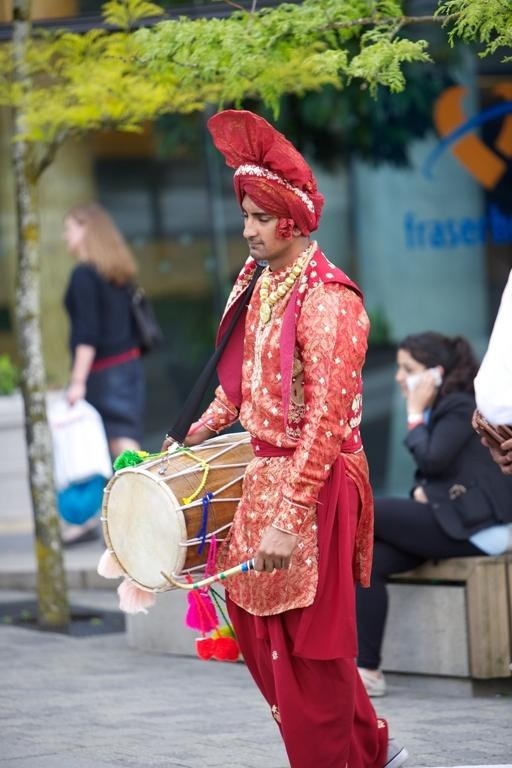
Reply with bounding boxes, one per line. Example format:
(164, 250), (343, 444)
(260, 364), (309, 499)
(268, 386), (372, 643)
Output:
(100, 432), (255, 593)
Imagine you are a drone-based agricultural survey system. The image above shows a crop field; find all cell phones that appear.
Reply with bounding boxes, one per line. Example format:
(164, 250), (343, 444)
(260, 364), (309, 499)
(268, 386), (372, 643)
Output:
(407, 368), (442, 392)
(475, 409), (512, 451)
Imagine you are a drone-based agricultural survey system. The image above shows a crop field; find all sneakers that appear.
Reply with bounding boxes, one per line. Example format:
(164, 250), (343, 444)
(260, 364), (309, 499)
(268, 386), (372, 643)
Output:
(384, 736), (408, 767)
(355, 666), (385, 697)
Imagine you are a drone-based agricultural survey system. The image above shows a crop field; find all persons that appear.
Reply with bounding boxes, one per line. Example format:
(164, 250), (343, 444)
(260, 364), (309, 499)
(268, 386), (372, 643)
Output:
(159, 110), (409, 768)
(355, 330), (512, 699)
(62, 200), (167, 546)
(471, 261), (512, 481)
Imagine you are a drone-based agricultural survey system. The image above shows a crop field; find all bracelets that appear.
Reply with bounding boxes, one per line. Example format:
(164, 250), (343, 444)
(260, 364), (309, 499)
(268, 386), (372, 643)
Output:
(407, 413), (424, 424)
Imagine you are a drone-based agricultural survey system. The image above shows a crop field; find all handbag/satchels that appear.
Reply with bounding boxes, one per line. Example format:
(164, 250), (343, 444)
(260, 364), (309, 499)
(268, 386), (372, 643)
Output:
(131, 286), (163, 346)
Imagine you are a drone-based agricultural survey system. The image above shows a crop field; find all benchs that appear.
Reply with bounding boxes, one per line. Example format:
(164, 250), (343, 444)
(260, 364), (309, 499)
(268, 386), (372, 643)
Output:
(120, 527), (512, 703)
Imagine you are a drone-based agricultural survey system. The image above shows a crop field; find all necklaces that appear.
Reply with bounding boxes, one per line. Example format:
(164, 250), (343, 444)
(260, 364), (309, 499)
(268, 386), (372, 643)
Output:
(257, 247), (312, 323)
